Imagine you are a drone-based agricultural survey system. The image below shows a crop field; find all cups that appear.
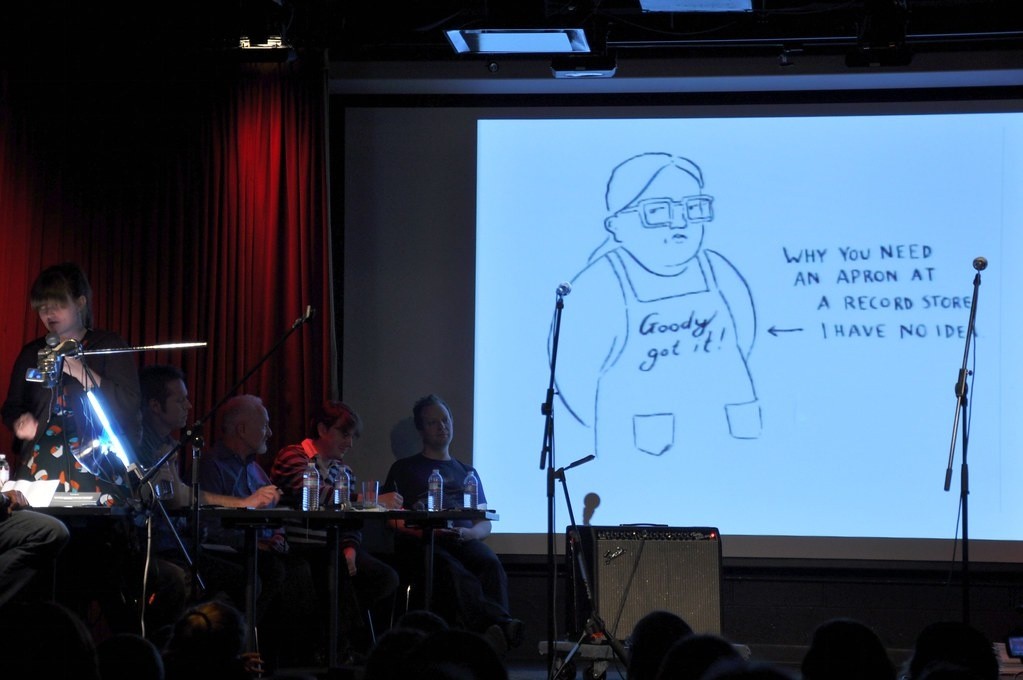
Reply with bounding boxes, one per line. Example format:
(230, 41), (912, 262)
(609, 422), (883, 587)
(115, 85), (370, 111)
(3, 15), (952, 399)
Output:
(362, 481), (379, 508)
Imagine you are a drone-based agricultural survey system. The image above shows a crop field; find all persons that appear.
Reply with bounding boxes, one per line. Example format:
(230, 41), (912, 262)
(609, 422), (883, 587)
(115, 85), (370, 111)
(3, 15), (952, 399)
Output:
(166, 600), (266, 680)
(269, 398), (401, 616)
(801, 619), (897, 680)
(198, 393), (316, 622)
(97, 632), (167, 680)
(0, 600), (103, 680)
(624, 608), (797, 680)
(386, 394), (525, 661)
(0, 497), (71, 606)
(0, 261), (142, 506)
(130, 367), (285, 599)
(360, 609), (508, 680)
(906, 619), (1000, 680)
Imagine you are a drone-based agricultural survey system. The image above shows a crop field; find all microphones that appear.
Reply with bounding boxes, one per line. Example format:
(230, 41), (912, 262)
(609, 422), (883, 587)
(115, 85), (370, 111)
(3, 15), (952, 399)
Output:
(556, 282), (573, 296)
(973, 257), (989, 271)
(46, 333), (63, 392)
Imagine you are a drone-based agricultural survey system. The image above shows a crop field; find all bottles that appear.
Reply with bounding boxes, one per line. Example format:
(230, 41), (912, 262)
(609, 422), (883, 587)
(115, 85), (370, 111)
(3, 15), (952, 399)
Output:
(463, 472), (479, 509)
(302, 463), (320, 511)
(154, 452), (173, 498)
(427, 470), (443, 512)
(0, 454), (10, 483)
(335, 467), (350, 506)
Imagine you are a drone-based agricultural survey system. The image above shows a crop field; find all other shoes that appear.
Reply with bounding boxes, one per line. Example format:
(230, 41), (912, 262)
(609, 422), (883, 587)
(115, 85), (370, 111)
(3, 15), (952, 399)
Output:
(312, 646), (368, 665)
(484, 624), (506, 657)
(506, 619), (525, 648)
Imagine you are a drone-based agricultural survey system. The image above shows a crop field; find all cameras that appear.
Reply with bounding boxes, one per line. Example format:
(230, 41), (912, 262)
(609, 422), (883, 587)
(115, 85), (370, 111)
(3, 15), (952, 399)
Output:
(25, 348), (64, 388)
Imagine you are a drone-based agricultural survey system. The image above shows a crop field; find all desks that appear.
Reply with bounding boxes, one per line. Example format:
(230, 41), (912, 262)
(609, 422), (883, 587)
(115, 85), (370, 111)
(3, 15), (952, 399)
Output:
(0, 502), (497, 680)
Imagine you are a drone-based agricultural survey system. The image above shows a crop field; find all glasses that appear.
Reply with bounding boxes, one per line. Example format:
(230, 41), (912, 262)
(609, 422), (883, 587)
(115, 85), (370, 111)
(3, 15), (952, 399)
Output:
(331, 426), (360, 440)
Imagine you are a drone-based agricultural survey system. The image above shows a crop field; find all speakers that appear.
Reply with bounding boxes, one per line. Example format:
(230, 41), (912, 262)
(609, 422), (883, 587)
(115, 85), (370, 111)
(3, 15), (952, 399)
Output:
(565, 524), (723, 647)
